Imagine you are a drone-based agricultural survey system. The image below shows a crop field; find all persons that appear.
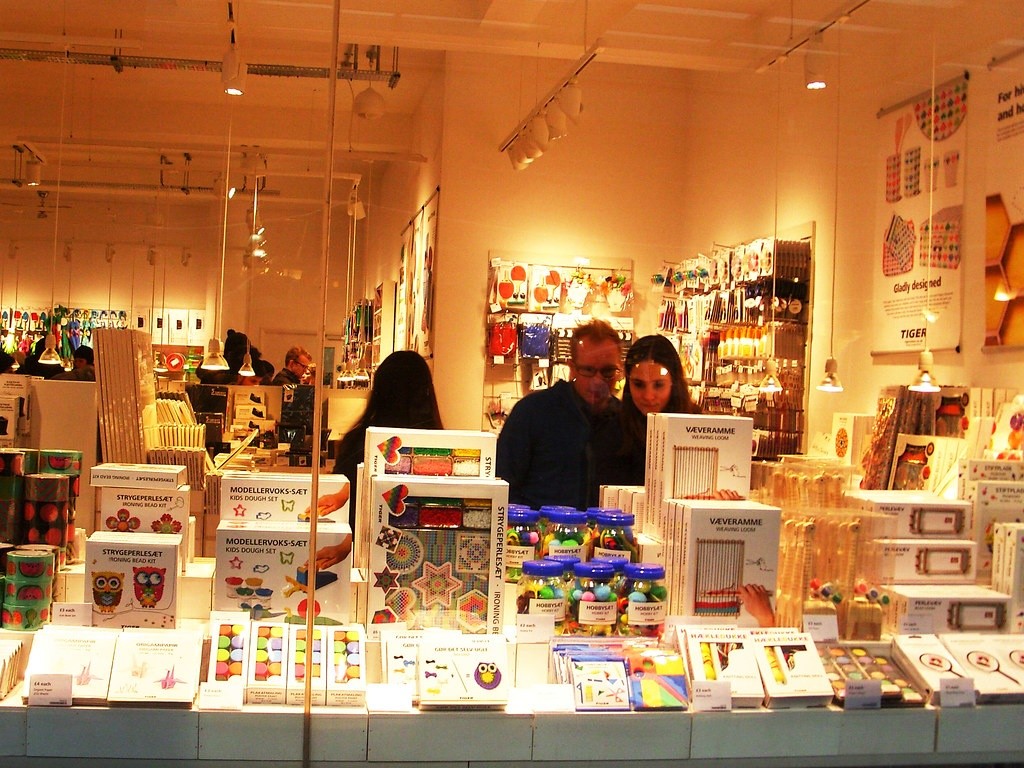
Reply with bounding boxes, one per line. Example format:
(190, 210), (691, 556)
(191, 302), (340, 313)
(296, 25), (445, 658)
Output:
(13, 338), (66, 378)
(304, 533), (352, 570)
(332, 351), (446, 542)
(242, 360), (275, 386)
(270, 345), (312, 386)
(305, 482), (350, 517)
(714, 489), (743, 500)
(621, 335), (703, 487)
(495, 322), (643, 513)
(737, 584), (775, 628)
(195, 328), (250, 385)
(51, 345), (95, 382)
(302, 363), (325, 386)
(0, 345), (15, 374)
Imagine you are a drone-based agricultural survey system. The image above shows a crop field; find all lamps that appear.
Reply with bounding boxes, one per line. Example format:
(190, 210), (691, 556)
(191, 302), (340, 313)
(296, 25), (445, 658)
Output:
(508, 76), (587, 172)
(818, 30), (842, 389)
(338, 180), (378, 382)
(220, 42), (248, 97)
(802, 27), (828, 91)
(355, 80), (387, 119)
(9, 141), (275, 376)
(757, 74), (783, 393)
(905, 36), (943, 393)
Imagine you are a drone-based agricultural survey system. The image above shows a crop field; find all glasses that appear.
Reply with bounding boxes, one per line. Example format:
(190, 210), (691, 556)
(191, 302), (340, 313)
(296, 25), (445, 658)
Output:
(574, 363), (621, 379)
(295, 360), (309, 369)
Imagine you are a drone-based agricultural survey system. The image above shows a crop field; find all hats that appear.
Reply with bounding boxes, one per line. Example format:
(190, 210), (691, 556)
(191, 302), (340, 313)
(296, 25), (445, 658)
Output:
(74, 346), (93, 364)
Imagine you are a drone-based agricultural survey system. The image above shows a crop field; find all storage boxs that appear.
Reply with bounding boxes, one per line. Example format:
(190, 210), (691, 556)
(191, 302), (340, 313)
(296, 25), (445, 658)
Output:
(0, 373), (43, 433)
(369, 427), (509, 708)
(29, 379), (99, 533)
(91, 462), (189, 488)
(100, 484), (192, 571)
(598, 386), (1024, 704)
(208, 473), (365, 708)
(85, 529), (183, 629)
(0, 395), (21, 450)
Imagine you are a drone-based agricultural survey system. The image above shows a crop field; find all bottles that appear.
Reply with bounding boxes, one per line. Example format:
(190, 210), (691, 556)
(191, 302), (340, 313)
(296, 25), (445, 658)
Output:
(337, 358), (369, 390)
(505, 503), (668, 638)
